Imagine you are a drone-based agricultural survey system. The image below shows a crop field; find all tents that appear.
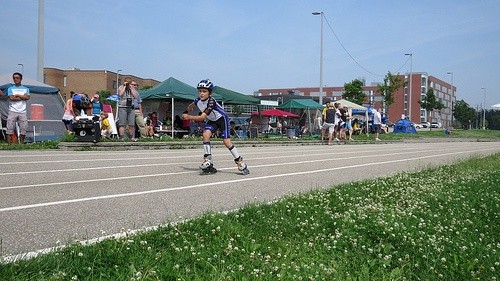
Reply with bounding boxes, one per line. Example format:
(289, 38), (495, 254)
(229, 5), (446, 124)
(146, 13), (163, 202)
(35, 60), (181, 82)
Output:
(0, 77), (417, 143)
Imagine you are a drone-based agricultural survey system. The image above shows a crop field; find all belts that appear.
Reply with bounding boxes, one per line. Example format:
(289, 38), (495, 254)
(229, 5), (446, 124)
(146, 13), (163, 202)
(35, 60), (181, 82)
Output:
(134, 108), (139, 109)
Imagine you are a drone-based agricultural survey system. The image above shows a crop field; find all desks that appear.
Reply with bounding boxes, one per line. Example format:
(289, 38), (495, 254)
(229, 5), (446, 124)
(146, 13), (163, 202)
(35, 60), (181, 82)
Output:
(27, 120), (62, 142)
(157, 130), (191, 132)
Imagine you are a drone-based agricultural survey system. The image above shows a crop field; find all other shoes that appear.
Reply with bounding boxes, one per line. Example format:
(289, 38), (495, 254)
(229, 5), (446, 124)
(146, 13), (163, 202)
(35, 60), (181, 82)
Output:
(120, 137), (124, 142)
(334, 138), (340, 141)
(349, 138), (354, 140)
(375, 138), (380, 141)
(131, 138), (138, 141)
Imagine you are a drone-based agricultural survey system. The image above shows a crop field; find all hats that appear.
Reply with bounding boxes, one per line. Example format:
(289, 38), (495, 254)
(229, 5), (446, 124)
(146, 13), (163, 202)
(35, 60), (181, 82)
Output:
(325, 101), (330, 104)
(94, 94), (99, 98)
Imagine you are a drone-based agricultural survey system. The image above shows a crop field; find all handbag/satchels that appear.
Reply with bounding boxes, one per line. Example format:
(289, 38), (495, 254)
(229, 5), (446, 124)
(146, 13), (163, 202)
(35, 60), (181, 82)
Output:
(340, 117), (345, 126)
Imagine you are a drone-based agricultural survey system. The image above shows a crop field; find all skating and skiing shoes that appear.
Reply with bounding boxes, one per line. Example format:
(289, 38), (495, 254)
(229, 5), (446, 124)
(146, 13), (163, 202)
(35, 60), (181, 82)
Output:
(234, 156), (250, 174)
(199, 153), (217, 174)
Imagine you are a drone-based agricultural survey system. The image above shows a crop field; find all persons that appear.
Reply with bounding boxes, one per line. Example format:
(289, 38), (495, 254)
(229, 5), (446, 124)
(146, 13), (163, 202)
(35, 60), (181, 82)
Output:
(6, 73), (30, 145)
(277, 101), (381, 145)
(182, 80), (249, 174)
(62, 76), (205, 142)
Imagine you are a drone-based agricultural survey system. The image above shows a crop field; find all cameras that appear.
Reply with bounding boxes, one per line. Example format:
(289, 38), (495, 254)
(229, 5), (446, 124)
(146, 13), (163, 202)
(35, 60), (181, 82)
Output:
(126, 83), (130, 86)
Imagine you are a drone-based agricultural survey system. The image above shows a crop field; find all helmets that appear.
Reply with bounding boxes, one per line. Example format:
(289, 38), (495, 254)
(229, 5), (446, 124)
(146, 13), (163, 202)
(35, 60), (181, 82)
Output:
(197, 80), (213, 92)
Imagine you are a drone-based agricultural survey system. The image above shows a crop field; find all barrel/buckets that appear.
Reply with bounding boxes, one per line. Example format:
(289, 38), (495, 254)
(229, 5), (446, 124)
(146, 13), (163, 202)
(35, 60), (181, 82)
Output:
(287, 126), (296, 138)
(30, 104), (44, 120)
